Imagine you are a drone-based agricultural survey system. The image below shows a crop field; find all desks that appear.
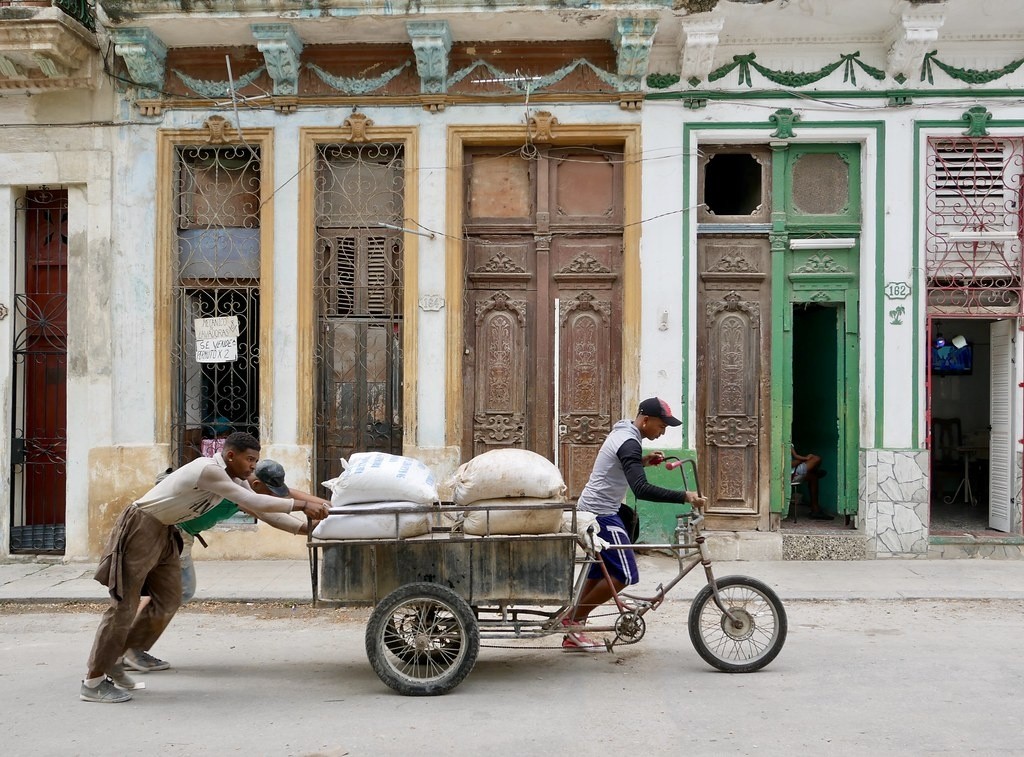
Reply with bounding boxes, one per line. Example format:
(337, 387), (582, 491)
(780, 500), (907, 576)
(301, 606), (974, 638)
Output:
(943, 443), (989, 507)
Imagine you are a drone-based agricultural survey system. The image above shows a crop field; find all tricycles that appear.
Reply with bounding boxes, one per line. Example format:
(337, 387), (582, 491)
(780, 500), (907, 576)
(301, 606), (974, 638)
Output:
(305, 450), (788, 698)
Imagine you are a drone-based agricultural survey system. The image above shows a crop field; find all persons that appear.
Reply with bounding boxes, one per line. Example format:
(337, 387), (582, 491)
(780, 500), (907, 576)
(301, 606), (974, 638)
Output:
(79, 432), (329, 703)
(558, 396), (707, 652)
(791, 443), (834, 520)
(106, 459), (332, 689)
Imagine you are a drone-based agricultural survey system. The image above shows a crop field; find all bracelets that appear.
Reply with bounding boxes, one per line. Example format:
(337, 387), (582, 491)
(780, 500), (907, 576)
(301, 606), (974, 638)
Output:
(301, 501), (309, 511)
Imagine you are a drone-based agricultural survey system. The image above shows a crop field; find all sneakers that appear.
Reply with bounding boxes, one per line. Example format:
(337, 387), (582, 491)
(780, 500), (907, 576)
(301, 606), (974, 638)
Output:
(79, 662), (135, 703)
(557, 611), (609, 653)
(116, 647), (170, 671)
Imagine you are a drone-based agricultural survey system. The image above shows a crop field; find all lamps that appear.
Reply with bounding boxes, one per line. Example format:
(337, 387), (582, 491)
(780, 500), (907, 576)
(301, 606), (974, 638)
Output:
(952, 334), (968, 349)
(937, 333), (945, 346)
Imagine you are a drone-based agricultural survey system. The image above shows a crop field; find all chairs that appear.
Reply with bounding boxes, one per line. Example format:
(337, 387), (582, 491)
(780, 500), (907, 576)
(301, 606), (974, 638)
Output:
(932, 418), (975, 498)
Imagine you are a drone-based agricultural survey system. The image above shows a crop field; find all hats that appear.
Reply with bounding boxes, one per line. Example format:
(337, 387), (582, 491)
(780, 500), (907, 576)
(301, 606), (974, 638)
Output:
(254, 459), (290, 497)
(638, 397), (683, 426)
